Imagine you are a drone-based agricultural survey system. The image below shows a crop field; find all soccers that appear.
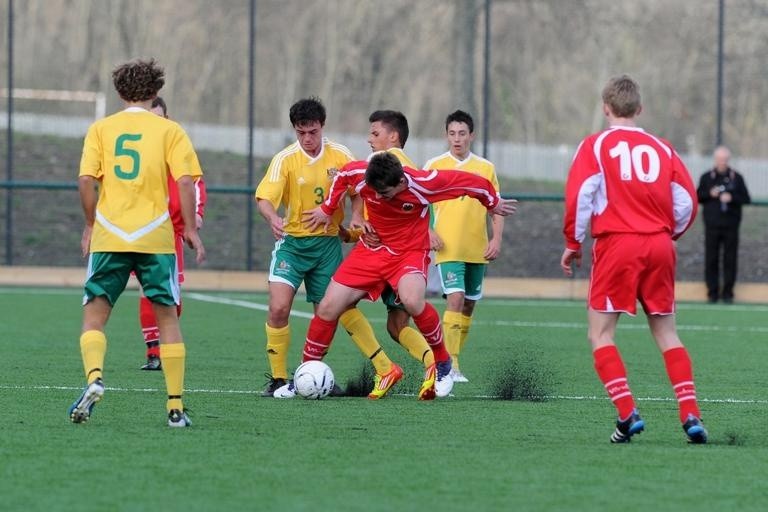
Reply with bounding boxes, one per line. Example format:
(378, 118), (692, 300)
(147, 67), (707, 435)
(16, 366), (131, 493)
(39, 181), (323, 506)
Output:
(294, 360), (335, 399)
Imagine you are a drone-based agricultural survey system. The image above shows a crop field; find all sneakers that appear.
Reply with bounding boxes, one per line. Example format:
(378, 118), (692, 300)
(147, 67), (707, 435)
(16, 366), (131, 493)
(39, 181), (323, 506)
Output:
(609, 407), (645, 445)
(167, 406), (192, 430)
(68, 380), (105, 424)
(273, 377), (298, 399)
(140, 353), (163, 371)
(367, 363), (404, 399)
(434, 355), (454, 399)
(328, 380), (345, 397)
(704, 295), (734, 306)
(417, 358), (436, 401)
(681, 414), (708, 443)
(452, 368), (470, 383)
(260, 377), (286, 397)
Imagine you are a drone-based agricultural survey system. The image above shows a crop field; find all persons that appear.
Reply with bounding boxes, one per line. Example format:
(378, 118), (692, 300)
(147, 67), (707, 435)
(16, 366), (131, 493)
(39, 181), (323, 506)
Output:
(560, 71), (713, 445)
(339, 109), (437, 398)
(128, 98), (206, 371)
(696, 144), (751, 303)
(419, 108), (504, 384)
(70, 56), (200, 428)
(255, 96), (375, 394)
(298, 151), (518, 398)
